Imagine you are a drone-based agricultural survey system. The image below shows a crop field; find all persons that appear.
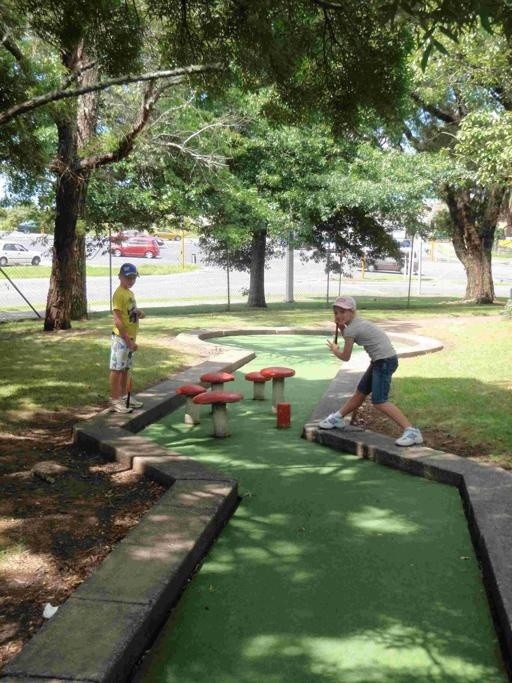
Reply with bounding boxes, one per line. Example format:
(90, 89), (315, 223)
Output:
(106, 261), (147, 414)
(317, 293), (424, 448)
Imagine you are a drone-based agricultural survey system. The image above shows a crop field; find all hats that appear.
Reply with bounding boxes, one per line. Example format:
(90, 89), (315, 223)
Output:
(332, 296), (358, 312)
(119, 263), (141, 278)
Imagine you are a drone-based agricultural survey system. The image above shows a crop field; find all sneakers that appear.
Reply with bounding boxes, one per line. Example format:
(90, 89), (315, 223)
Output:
(319, 412), (346, 429)
(111, 394), (143, 412)
(394, 428), (425, 446)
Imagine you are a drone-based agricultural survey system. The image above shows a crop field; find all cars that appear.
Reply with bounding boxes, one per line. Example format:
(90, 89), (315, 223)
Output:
(0, 241), (42, 266)
(109, 227), (182, 259)
(17, 220), (55, 233)
(355, 238), (419, 274)
